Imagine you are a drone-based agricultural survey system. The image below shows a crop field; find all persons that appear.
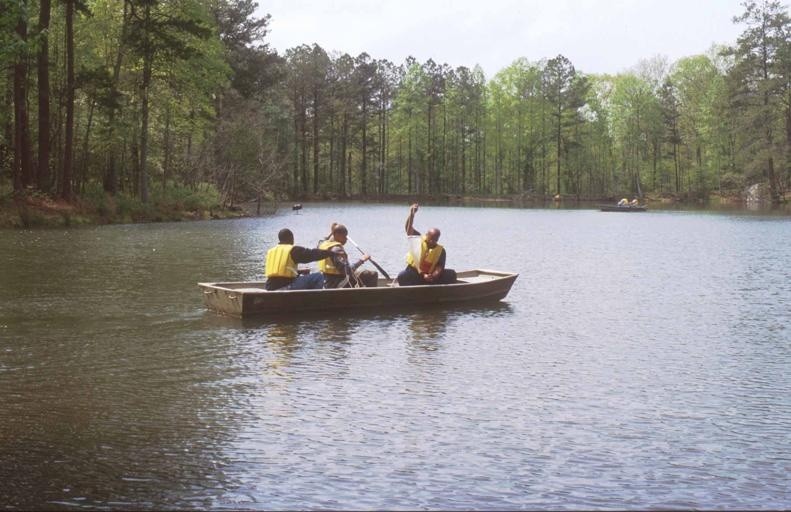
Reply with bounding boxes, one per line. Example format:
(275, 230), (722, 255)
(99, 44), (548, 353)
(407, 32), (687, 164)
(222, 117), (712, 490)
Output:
(317, 222), (379, 289)
(264, 227), (349, 290)
(396, 202), (458, 285)
(618, 195), (629, 207)
(629, 195), (640, 206)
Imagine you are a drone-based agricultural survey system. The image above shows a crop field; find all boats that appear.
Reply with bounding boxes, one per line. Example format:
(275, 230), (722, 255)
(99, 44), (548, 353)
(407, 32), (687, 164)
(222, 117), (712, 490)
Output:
(193, 268), (520, 322)
(597, 203), (650, 213)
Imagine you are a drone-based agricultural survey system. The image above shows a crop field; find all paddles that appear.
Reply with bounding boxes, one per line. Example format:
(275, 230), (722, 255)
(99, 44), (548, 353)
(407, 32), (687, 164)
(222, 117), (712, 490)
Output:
(346, 235), (391, 281)
